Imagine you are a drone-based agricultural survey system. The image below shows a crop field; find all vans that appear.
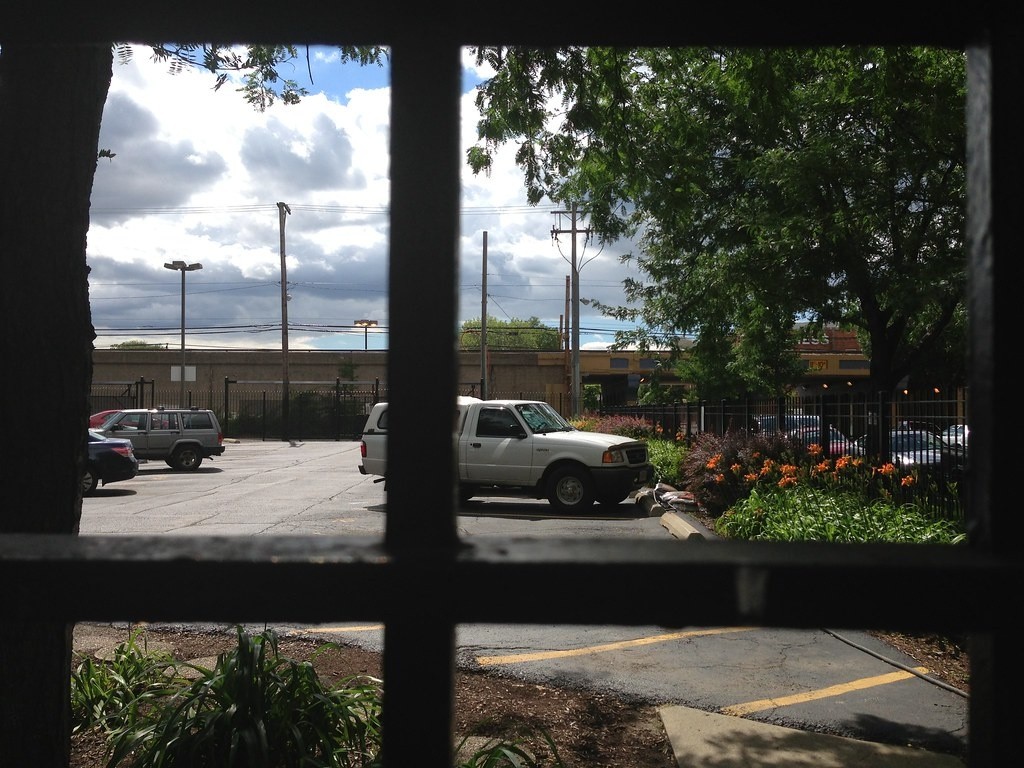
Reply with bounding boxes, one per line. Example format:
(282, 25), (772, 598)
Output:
(88, 403), (226, 472)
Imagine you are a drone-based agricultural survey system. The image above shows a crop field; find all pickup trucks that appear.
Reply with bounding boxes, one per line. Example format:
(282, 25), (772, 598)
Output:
(358, 396), (654, 515)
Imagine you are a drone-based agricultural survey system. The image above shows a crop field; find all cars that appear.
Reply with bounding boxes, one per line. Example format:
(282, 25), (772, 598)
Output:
(89, 409), (178, 429)
(753, 411), (852, 466)
(83, 430), (138, 498)
(936, 424), (970, 450)
(854, 426), (962, 470)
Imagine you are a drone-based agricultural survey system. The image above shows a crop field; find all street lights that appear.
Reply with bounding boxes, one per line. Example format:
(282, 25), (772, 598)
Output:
(353, 319), (379, 350)
(164, 261), (203, 408)
